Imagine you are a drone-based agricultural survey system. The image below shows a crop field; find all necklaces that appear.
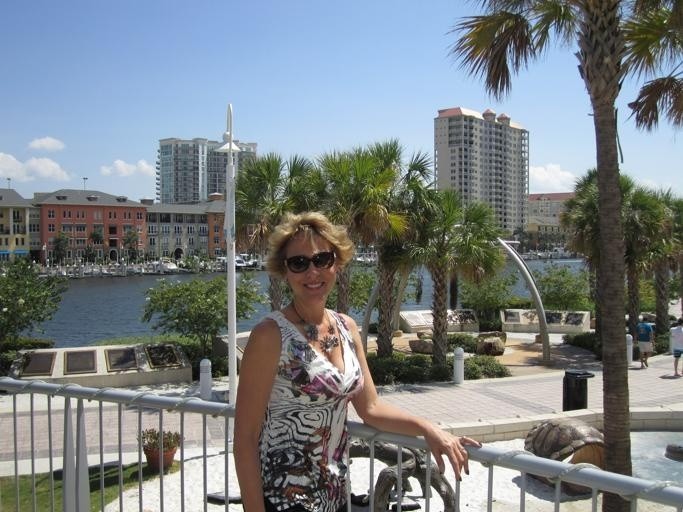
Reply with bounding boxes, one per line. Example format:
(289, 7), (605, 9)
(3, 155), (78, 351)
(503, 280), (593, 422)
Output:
(291, 299), (339, 354)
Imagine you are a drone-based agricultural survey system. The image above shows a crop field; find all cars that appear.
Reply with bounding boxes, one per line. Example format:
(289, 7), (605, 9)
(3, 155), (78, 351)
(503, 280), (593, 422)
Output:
(155, 251), (262, 274)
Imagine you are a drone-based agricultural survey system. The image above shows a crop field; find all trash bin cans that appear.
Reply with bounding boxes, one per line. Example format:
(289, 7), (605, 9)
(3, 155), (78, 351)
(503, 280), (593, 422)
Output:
(560, 369), (595, 411)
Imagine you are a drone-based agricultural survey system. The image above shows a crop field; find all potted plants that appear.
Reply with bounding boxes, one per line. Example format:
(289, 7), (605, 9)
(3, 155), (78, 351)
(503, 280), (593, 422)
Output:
(138, 429), (182, 471)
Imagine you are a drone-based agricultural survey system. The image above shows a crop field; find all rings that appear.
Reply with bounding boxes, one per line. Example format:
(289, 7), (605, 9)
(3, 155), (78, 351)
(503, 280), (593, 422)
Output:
(461, 436), (467, 444)
(460, 448), (465, 452)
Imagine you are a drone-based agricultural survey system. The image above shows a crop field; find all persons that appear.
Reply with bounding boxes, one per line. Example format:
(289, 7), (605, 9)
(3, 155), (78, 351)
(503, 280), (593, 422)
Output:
(636, 315), (655, 368)
(669, 318), (683, 377)
(234, 211), (481, 512)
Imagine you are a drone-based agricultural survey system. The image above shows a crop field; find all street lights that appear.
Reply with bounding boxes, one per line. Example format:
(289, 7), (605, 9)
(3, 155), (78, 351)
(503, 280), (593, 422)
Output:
(31, 257), (152, 274)
(216, 104), (241, 407)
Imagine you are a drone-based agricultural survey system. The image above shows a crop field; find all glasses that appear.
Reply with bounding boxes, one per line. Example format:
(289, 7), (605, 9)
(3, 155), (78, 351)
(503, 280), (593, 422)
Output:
(285, 249), (335, 273)
